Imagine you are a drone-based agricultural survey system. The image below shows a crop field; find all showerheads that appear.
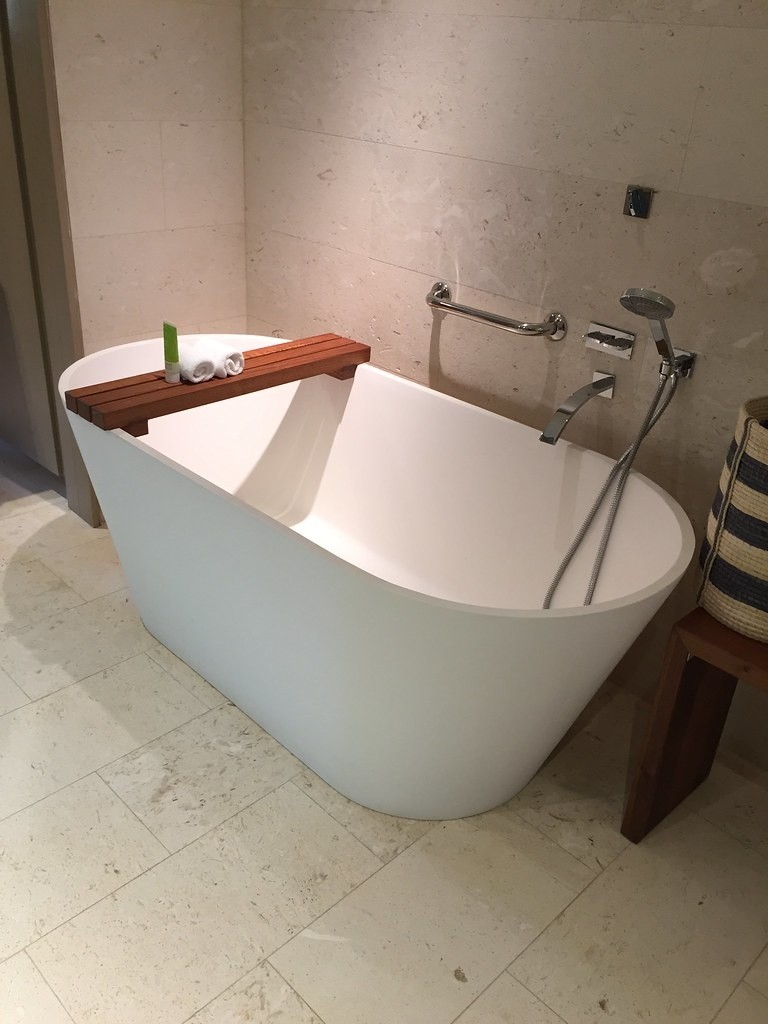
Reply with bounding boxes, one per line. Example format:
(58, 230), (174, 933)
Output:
(619, 286), (677, 376)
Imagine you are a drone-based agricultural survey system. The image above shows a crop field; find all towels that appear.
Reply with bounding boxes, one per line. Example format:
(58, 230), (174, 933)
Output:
(176, 334), (246, 383)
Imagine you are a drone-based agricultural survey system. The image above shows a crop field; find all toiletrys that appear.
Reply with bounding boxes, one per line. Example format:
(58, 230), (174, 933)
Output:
(161, 317), (181, 384)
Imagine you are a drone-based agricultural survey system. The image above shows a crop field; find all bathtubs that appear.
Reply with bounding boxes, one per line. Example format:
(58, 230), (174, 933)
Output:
(58, 331), (697, 821)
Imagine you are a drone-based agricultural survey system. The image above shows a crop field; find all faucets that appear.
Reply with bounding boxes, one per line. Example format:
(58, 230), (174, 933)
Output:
(539, 368), (617, 447)
